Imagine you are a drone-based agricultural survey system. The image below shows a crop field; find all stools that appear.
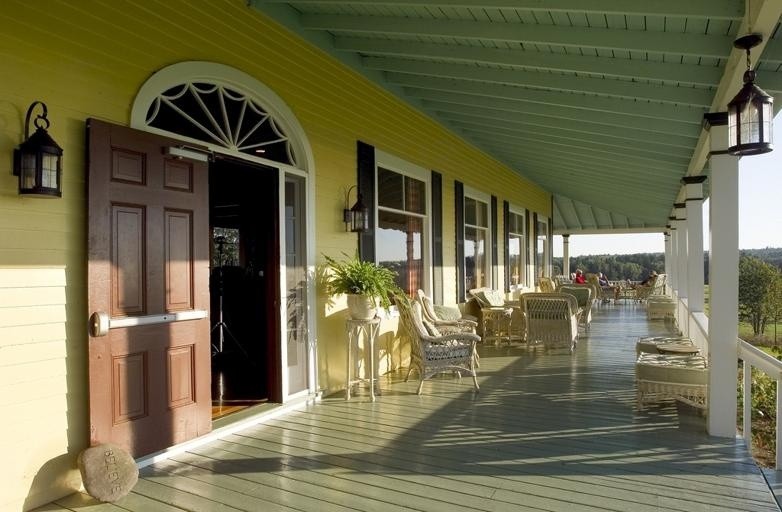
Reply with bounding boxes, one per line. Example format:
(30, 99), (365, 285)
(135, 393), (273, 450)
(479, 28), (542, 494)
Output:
(637, 352), (710, 415)
(648, 300), (676, 321)
(636, 337), (696, 383)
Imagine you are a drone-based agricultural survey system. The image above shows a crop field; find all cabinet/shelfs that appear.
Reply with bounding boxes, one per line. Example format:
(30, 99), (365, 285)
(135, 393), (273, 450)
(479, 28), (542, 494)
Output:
(345, 318), (383, 403)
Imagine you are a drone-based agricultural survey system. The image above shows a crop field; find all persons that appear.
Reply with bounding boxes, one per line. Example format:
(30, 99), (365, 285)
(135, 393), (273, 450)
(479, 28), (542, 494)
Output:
(572, 266), (657, 302)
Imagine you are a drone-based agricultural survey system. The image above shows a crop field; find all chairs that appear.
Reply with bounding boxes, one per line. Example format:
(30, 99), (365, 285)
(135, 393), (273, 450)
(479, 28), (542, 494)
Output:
(469, 271), (603, 357)
(395, 289), (481, 394)
(582, 272), (669, 311)
(413, 289), (480, 378)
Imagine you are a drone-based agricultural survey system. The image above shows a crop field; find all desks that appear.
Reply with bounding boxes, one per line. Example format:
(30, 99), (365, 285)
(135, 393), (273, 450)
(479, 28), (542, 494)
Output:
(657, 344), (700, 355)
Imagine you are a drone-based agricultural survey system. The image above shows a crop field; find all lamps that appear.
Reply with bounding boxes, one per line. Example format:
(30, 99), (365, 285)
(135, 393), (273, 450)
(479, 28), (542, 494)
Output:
(12, 101), (62, 200)
(728, 32), (775, 157)
(343, 184), (369, 233)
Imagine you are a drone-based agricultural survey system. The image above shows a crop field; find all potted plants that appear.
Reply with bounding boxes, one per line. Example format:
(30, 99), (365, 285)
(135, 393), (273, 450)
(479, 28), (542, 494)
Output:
(318, 248), (411, 321)
